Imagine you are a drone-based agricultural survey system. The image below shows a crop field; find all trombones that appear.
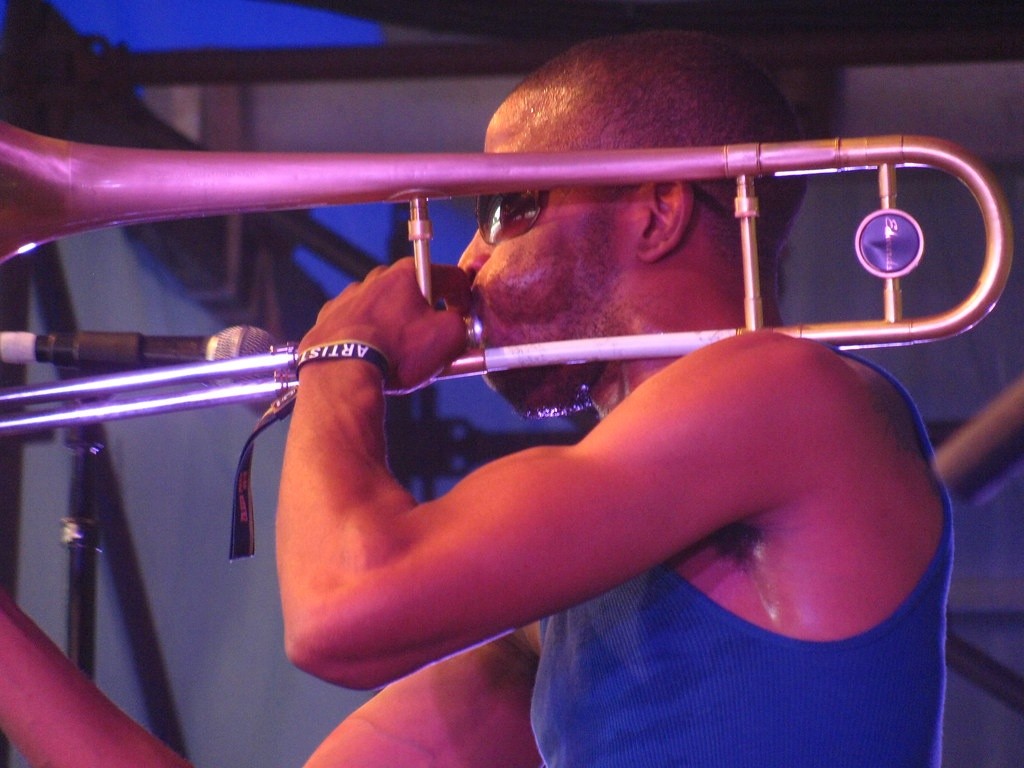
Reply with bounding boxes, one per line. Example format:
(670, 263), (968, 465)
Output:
(0, 113), (1012, 435)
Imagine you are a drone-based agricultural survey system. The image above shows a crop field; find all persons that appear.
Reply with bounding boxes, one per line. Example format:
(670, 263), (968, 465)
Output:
(0, 29), (958, 767)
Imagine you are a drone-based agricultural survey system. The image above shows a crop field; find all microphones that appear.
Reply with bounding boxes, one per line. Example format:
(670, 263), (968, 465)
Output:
(0, 324), (277, 373)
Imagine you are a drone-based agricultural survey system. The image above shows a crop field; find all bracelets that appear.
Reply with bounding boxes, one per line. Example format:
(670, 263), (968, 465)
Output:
(295, 339), (388, 379)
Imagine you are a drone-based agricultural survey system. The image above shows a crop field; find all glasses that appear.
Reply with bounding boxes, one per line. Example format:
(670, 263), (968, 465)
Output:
(475, 190), (547, 246)
